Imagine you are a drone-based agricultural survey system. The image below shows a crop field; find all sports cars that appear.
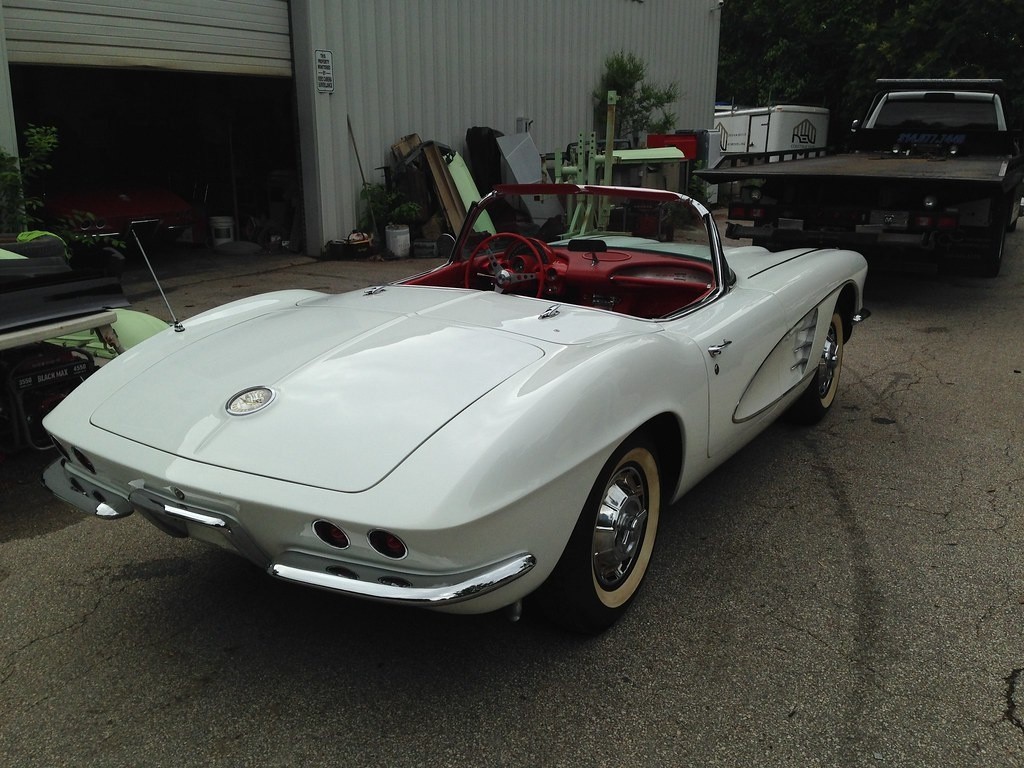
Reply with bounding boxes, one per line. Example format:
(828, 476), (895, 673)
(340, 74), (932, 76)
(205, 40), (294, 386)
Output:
(38, 182), (871, 629)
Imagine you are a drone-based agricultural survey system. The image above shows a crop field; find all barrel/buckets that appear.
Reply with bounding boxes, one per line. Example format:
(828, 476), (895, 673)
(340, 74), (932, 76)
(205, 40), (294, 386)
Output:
(386, 226), (410, 258)
(209, 216), (235, 248)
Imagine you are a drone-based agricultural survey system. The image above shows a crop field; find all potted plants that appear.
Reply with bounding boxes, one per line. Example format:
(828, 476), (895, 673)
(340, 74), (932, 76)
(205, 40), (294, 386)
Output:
(360, 184), (423, 259)
(685, 160), (715, 224)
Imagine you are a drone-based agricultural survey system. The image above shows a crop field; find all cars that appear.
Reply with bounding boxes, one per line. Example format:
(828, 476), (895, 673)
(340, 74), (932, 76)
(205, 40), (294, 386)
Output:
(42, 179), (197, 252)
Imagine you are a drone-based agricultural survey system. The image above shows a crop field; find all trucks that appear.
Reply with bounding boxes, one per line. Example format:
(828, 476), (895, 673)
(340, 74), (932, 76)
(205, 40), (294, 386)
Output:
(691, 77), (1024, 280)
